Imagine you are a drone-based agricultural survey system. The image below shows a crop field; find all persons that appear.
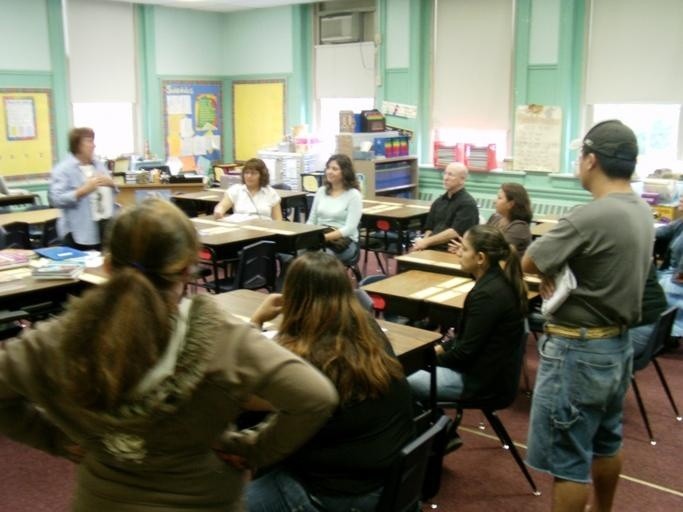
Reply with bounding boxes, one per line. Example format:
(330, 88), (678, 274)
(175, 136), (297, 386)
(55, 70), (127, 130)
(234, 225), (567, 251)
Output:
(234, 251), (410, 511)
(520, 120), (656, 511)
(626, 188), (683, 363)
(404, 224), (525, 458)
(405, 163), (480, 253)
(448, 182), (532, 263)
(212, 157), (284, 291)
(276, 155), (365, 294)
(48, 128), (119, 251)
(0, 199), (340, 511)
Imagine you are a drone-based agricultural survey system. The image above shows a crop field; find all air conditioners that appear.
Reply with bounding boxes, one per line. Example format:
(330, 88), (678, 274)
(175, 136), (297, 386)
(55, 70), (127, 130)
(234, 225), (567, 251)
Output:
(320, 15), (356, 43)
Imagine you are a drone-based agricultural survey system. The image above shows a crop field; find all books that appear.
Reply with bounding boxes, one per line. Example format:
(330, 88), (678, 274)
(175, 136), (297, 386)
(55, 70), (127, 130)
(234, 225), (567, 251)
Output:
(1, 248), (88, 283)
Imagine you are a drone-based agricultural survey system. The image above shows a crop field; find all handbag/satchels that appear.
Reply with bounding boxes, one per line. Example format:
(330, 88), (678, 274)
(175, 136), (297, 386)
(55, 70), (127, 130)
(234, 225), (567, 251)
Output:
(325, 228), (352, 254)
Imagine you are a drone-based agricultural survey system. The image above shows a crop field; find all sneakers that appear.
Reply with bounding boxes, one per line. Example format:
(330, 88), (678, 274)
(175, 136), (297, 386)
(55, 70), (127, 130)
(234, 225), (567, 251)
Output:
(445, 432), (463, 453)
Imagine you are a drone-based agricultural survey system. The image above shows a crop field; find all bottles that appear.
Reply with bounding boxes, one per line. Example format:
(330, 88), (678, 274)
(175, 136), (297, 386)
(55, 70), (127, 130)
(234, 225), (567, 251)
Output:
(339, 110), (356, 133)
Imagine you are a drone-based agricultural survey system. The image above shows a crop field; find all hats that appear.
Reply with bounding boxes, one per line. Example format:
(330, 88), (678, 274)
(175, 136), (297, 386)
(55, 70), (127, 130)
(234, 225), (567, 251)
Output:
(572, 119), (638, 161)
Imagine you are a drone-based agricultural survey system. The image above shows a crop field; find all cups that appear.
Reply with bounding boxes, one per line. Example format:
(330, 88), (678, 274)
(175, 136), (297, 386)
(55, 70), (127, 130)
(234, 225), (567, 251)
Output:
(151, 168), (162, 184)
(137, 168), (150, 184)
